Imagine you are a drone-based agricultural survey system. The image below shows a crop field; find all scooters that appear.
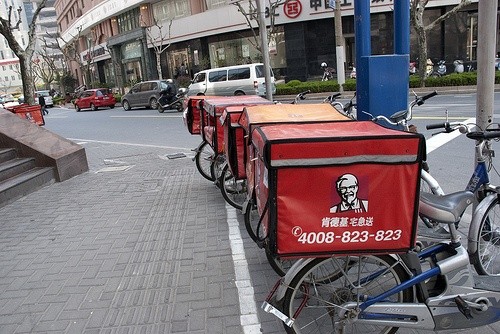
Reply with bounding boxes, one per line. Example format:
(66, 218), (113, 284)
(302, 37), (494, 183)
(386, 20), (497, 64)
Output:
(453, 59), (464, 74)
(436, 59), (447, 76)
(409, 61), (416, 76)
(182, 88), (500, 334)
(424, 58), (434, 77)
(349, 66), (356, 79)
(321, 62), (334, 81)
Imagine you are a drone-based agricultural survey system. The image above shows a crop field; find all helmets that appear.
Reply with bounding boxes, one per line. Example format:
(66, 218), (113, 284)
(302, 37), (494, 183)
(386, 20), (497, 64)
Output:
(166, 79), (173, 83)
(321, 62), (327, 67)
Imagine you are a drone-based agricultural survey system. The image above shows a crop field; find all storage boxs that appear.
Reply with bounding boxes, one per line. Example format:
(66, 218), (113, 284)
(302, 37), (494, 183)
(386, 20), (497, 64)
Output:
(5, 103), (45, 126)
(181, 93), (427, 254)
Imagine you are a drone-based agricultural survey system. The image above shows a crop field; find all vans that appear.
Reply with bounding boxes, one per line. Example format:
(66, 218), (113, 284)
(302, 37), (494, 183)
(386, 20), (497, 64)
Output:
(182, 62), (277, 100)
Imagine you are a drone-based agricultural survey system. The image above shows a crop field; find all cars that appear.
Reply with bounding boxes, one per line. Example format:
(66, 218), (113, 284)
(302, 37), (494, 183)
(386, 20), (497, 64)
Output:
(120, 79), (175, 111)
(0, 89), (54, 111)
(73, 87), (116, 112)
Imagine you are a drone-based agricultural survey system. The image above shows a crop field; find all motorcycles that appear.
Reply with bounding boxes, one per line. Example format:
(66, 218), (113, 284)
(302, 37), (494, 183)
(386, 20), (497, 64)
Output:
(156, 87), (189, 113)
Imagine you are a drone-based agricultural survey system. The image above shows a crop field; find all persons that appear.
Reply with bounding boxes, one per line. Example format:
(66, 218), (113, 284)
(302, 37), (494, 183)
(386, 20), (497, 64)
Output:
(320, 62), (334, 81)
(159, 79), (176, 107)
(350, 67), (357, 79)
(495, 51), (500, 70)
(409, 63), (416, 75)
(38, 93), (48, 115)
